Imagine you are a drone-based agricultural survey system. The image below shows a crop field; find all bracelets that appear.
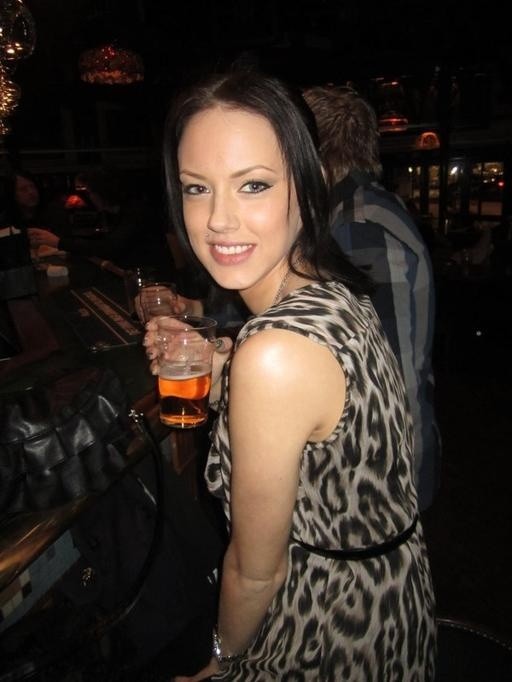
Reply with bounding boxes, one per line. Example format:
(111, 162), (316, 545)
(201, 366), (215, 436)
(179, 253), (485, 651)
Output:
(211, 629), (236, 665)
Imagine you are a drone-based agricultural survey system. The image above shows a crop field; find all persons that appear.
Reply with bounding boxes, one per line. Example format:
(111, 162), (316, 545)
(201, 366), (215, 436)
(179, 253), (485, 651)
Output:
(139, 60), (439, 681)
(0, 156), (144, 273)
(135, 71), (450, 517)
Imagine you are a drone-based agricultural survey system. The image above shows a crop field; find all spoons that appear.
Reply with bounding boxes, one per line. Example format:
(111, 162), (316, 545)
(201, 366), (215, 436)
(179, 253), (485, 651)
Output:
(88, 341), (139, 353)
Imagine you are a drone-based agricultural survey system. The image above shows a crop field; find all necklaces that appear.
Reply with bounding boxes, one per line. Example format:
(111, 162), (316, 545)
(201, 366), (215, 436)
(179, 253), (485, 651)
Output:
(271, 261), (301, 308)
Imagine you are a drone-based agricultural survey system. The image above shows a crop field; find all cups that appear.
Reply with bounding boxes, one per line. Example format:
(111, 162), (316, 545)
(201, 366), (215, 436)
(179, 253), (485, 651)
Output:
(156, 316), (218, 429)
(139, 282), (177, 323)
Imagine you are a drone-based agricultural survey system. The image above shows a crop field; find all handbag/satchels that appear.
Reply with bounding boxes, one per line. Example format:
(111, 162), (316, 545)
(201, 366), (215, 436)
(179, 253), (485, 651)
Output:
(0, 389), (132, 510)
